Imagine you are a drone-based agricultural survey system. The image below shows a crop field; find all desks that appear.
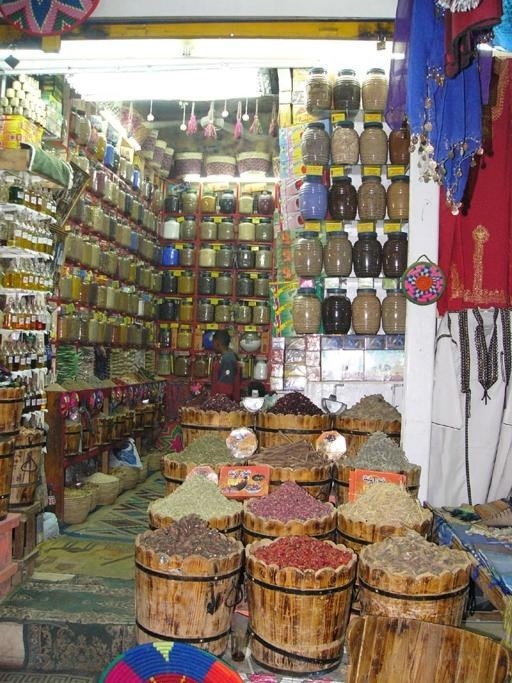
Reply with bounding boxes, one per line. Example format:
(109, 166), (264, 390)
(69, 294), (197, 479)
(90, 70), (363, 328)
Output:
(435, 496), (510, 619)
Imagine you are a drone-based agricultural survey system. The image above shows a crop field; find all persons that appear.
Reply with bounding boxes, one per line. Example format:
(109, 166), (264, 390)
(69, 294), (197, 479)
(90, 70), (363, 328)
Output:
(209, 326), (242, 400)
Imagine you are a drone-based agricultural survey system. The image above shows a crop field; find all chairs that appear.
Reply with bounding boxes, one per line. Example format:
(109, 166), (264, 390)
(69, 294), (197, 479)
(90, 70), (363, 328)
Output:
(346, 614), (509, 678)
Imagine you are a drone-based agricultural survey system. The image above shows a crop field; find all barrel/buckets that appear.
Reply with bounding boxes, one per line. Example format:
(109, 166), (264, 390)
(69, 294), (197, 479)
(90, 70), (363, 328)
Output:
(134, 396), (512, 683)
(0, 385), (160, 524)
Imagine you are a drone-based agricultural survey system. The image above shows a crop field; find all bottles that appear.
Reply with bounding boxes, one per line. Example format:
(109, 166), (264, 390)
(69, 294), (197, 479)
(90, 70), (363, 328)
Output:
(0, 68), (407, 411)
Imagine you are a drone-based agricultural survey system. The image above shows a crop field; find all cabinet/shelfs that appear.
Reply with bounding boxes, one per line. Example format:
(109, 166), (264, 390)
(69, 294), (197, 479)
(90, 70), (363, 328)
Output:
(0, 71), (410, 532)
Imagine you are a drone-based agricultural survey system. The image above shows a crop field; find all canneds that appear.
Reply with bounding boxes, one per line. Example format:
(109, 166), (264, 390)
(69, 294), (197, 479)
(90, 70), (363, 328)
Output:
(57, 107), (276, 381)
(284, 68), (409, 338)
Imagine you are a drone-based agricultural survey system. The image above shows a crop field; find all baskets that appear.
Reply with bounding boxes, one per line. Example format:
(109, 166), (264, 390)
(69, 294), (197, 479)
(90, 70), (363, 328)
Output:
(64, 454), (161, 524)
